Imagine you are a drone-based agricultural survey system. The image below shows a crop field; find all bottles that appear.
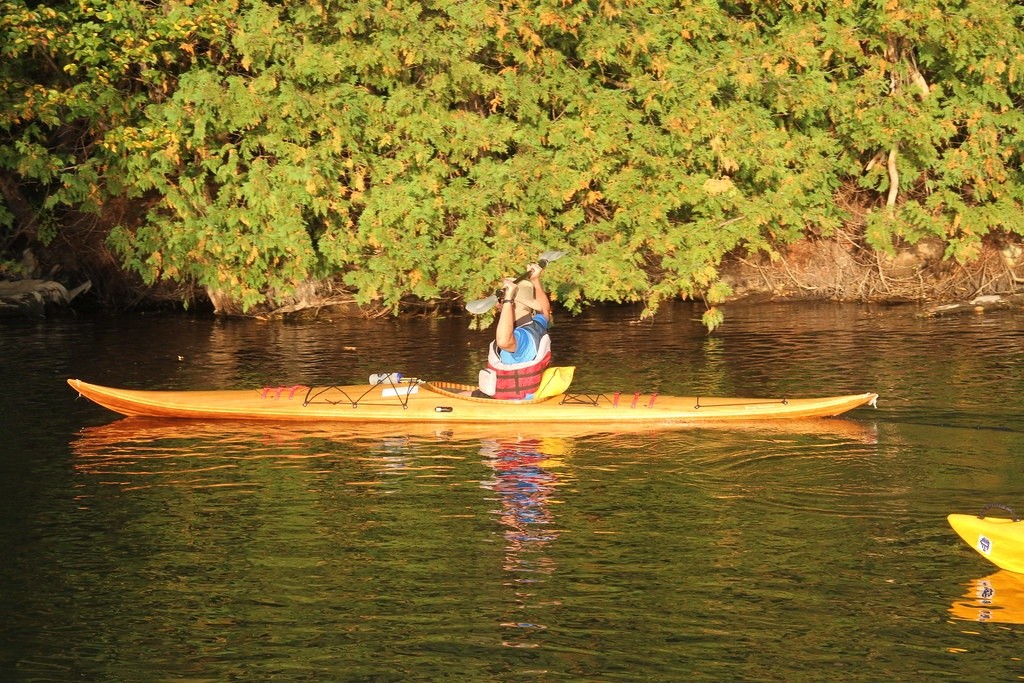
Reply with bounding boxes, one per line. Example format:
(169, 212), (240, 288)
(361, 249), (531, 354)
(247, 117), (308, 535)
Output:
(369, 373), (402, 385)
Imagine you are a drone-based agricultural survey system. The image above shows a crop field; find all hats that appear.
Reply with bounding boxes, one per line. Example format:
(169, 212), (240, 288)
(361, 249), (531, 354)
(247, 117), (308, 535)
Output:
(504, 278), (541, 312)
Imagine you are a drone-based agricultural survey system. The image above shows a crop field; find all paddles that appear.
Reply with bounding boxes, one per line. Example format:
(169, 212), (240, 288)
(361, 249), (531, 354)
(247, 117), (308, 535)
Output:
(465, 250), (570, 314)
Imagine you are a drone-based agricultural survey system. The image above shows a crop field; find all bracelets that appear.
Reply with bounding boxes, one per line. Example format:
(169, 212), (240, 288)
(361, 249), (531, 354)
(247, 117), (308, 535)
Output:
(503, 299), (514, 306)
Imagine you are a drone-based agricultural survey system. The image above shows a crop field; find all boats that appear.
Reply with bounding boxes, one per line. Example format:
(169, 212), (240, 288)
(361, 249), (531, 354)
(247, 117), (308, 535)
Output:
(951, 572), (1024, 626)
(67, 411), (880, 454)
(66, 375), (880, 424)
(948, 512), (1023, 573)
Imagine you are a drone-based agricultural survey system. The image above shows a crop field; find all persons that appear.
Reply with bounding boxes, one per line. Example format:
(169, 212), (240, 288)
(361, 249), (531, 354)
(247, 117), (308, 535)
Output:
(458, 263), (551, 400)
